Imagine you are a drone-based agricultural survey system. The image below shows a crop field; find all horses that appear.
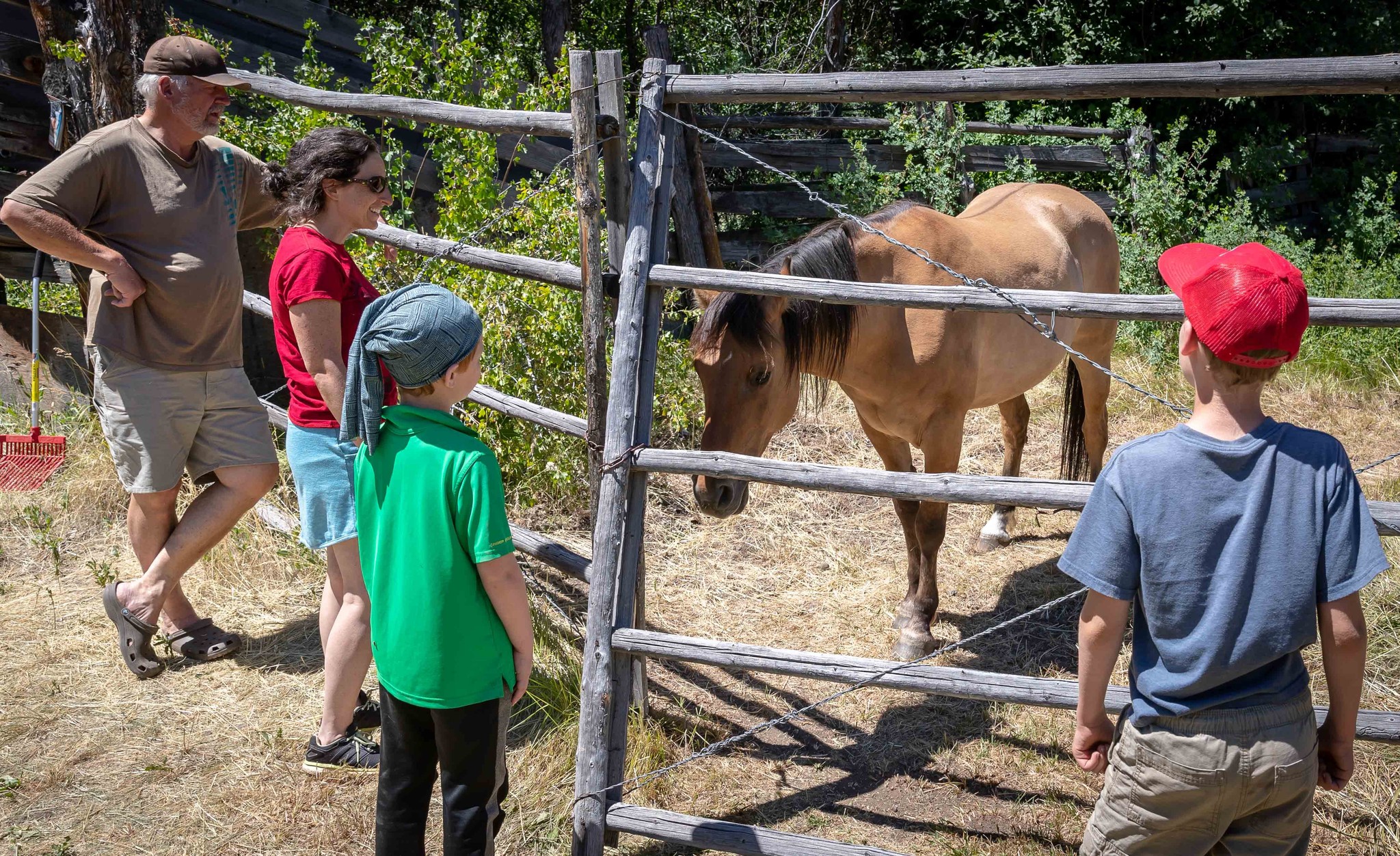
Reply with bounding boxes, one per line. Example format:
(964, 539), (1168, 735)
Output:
(684, 181), (1125, 663)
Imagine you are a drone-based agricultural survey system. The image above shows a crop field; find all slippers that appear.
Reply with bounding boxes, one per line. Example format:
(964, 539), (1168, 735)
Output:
(163, 618), (243, 661)
(102, 578), (163, 681)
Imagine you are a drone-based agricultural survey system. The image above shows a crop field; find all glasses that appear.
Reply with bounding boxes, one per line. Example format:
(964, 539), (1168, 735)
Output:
(340, 176), (389, 194)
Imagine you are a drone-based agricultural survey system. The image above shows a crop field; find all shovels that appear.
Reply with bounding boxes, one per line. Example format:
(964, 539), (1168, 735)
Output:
(0, 248), (66, 491)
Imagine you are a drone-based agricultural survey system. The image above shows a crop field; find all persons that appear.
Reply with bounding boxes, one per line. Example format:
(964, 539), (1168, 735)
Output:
(340, 284), (535, 856)
(0, 35), (397, 679)
(1057, 242), (1393, 856)
(269, 126), (399, 775)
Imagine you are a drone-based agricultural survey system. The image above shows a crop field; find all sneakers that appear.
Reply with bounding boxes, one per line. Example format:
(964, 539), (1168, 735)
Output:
(353, 690), (381, 729)
(301, 722), (381, 776)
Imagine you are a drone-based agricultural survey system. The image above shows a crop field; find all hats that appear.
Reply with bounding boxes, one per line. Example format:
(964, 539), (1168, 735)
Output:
(1156, 242), (1309, 368)
(144, 34), (251, 91)
